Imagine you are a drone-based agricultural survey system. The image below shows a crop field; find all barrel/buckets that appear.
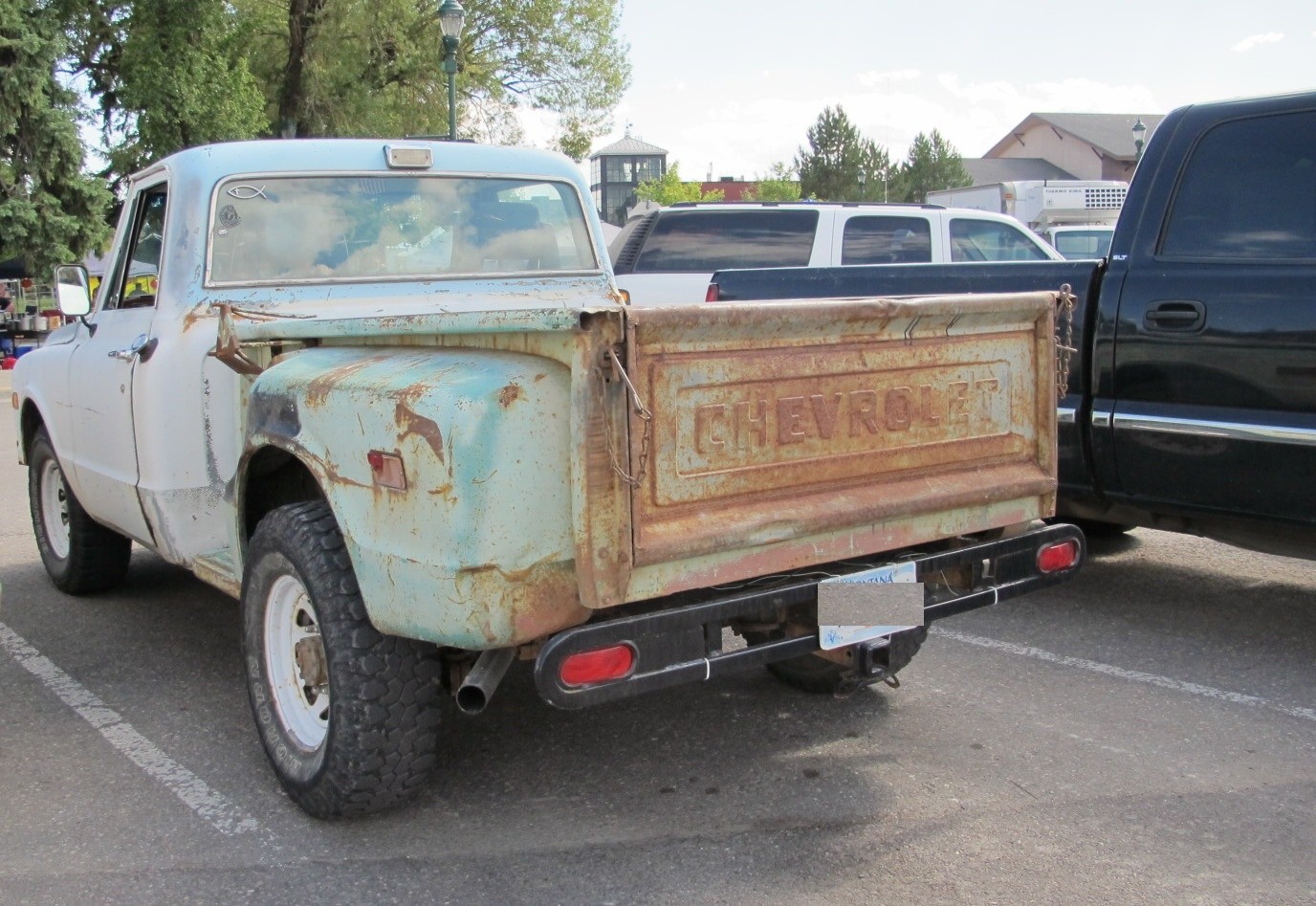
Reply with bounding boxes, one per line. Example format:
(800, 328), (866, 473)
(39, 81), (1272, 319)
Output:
(1, 338), (12, 350)
(49, 316), (61, 329)
(16, 344), (34, 358)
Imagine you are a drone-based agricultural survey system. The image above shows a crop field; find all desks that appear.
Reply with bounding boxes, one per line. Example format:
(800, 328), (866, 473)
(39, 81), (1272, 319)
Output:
(0, 329), (52, 358)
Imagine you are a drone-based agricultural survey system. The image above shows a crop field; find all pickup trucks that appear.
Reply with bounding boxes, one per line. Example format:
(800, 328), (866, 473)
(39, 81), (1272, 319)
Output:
(704, 91), (1315, 560)
(12, 138), (1090, 819)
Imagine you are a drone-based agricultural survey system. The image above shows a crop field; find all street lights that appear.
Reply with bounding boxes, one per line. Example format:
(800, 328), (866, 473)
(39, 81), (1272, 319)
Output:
(437, 0), (466, 142)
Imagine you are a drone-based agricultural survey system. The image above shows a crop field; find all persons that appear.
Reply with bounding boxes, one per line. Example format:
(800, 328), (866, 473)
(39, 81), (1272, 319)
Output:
(93, 276), (102, 307)
(0, 284), (15, 311)
(129, 283), (147, 296)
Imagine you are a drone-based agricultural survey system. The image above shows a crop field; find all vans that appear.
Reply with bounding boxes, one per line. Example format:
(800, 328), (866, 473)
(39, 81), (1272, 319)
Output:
(606, 201), (1070, 307)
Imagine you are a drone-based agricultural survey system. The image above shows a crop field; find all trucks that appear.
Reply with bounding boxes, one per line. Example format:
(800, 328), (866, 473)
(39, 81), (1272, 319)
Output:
(926, 180), (1140, 261)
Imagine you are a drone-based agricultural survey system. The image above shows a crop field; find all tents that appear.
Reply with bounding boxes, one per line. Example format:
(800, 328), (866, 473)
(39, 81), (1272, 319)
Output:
(61, 252), (157, 326)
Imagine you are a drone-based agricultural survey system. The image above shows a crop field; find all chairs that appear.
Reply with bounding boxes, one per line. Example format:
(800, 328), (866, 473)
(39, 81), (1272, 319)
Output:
(281, 204), (386, 279)
(449, 201), (563, 273)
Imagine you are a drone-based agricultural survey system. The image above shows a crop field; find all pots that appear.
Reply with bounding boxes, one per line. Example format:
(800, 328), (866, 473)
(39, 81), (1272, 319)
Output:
(6, 312), (48, 330)
